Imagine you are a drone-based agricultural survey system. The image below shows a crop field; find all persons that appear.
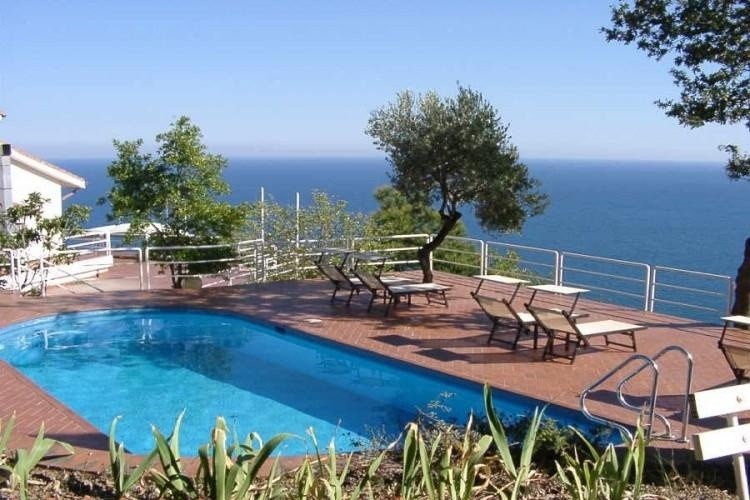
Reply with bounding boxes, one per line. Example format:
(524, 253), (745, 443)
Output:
(731, 237), (750, 332)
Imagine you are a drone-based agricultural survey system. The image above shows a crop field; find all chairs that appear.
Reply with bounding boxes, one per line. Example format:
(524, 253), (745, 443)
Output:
(717, 315), (750, 382)
(467, 273), (591, 349)
(316, 247), (415, 306)
(351, 252), (452, 319)
(524, 284), (649, 365)
